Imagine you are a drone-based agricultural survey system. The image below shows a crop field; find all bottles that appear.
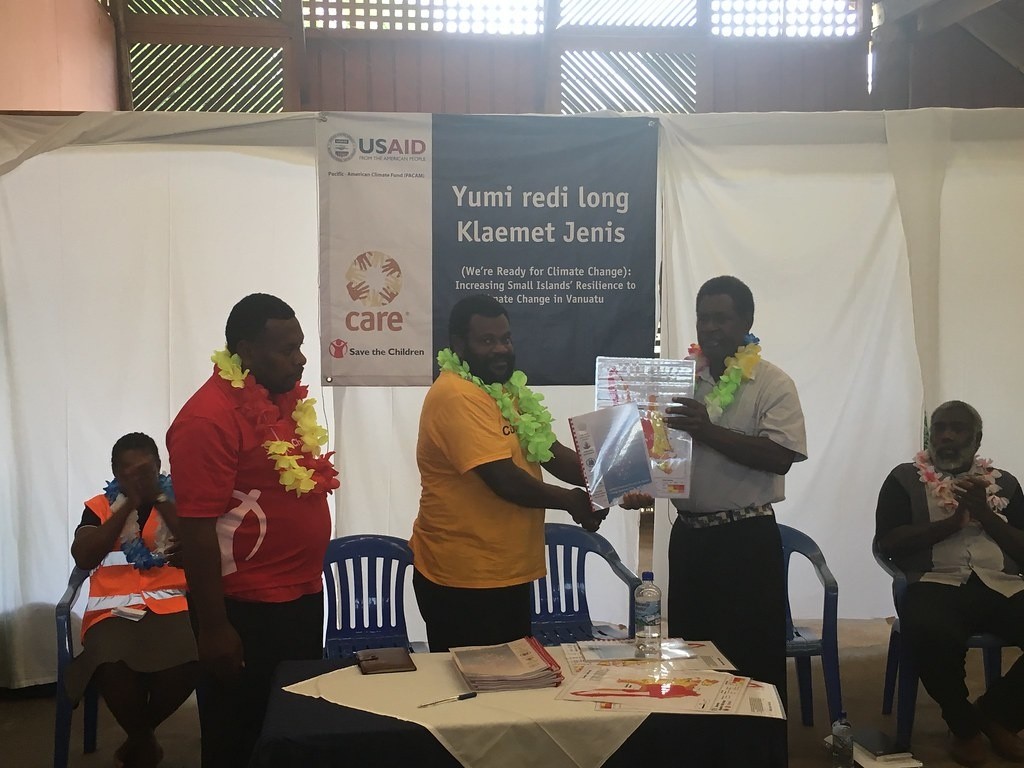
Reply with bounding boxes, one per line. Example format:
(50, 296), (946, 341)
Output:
(832, 710), (854, 768)
(634, 571), (662, 652)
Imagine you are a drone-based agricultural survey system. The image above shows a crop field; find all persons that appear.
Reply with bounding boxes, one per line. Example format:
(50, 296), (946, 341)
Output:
(407, 295), (614, 655)
(664, 276), (808, 715)
(166, 293), (340, 768)
(874, 402), (1024, 768)
(71, 431), (195, 768)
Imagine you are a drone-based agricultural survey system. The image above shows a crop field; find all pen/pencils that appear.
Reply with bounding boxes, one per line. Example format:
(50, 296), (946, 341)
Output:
(418, 692), (477, 708)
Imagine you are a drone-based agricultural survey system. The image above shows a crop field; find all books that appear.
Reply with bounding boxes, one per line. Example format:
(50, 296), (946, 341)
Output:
(355, 647), (417, 675)
(576, 639), (648, 661)
(448, 636), (565, 693)
(567, 402), (653, 515)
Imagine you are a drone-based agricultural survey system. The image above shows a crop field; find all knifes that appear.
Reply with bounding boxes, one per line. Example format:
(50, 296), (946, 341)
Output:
(418, 691), (477, 709)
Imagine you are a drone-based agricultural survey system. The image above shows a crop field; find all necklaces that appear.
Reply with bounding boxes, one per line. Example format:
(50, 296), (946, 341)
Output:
(103, 473), (176, 570)
(212, 347), (340, 499)
(914, 446), (1010, 524)
(434, 348), (556, 464)
(685, 333), (762, 423)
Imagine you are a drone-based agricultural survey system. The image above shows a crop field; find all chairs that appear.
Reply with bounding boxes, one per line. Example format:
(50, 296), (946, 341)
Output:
(54, 564), (205, 768)
(872, 533), (1016, 750)
(774, 522), (843, 726)
(527, 522), (640, 647)
(317, 535), (430, 658)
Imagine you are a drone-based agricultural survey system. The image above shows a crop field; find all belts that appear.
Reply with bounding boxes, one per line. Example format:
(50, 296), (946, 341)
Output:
(678, 501), (773, 529)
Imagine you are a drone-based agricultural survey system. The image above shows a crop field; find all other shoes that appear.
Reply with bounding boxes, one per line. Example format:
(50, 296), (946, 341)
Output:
(114, 728), (164, 768)
(945, 721), (986, 767)
(972, 694), (1024, 762)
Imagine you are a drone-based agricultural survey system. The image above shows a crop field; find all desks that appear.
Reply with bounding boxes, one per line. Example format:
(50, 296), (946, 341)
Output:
(246, 641), (789, 768)
